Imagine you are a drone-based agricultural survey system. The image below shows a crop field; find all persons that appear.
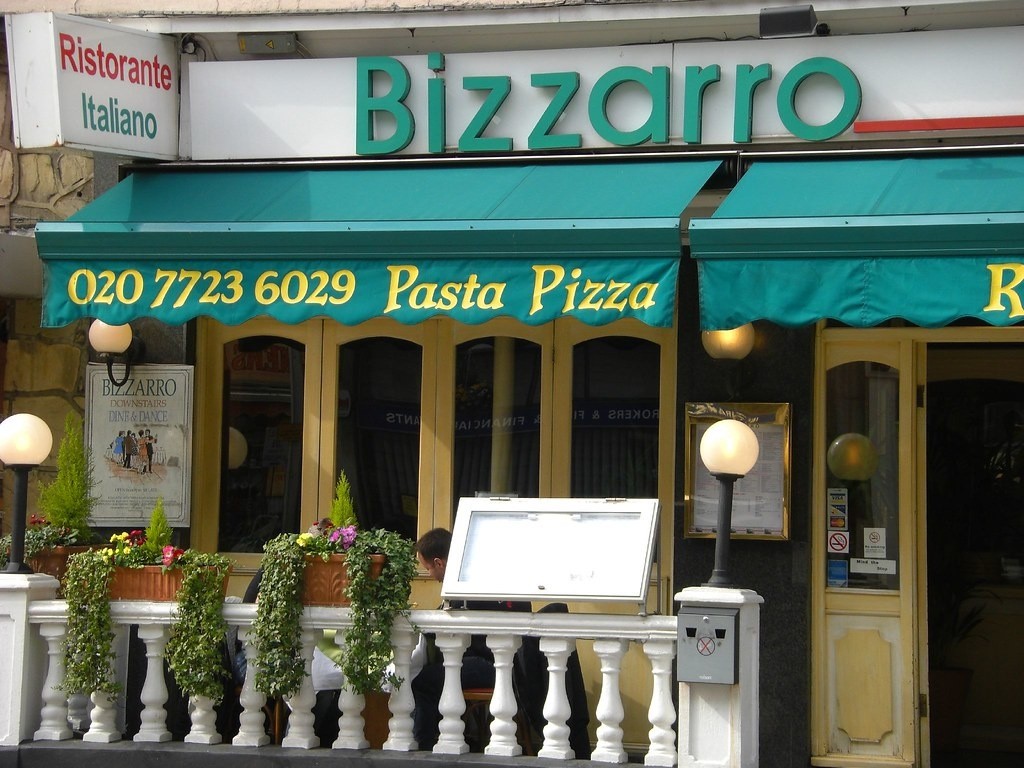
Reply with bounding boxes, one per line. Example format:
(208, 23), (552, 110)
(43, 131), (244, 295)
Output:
(415, 527), (518, 753)
(113, 429), (158, 475)
(235, 517), (339, 743)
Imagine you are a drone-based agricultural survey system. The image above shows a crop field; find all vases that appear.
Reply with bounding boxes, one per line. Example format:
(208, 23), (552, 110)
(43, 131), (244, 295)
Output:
(7, 545), (113, 577)
(300, 554), (386, 607)
(109, 564), (232, 602)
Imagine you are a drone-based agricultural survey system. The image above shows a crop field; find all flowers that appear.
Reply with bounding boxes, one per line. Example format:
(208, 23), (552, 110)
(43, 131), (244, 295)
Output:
(0, 411), (108, 568)
(242, 471), (425, 698)
(51, 498), (246, 706)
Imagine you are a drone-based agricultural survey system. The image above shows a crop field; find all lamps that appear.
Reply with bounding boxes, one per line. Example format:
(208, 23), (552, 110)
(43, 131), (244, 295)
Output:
(826, 433), (877, 586)
(89, 319), (143, 387)
(759, 4), (819, 38)
(701, 419), (759, 587)
(700, 322), (755, 403)
(0, 413), (53, 574)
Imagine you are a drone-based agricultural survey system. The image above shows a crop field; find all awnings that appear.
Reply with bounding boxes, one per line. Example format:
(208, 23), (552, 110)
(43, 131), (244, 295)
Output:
(34, 160), (724, 328)
(688, 157), (1024, 332)
